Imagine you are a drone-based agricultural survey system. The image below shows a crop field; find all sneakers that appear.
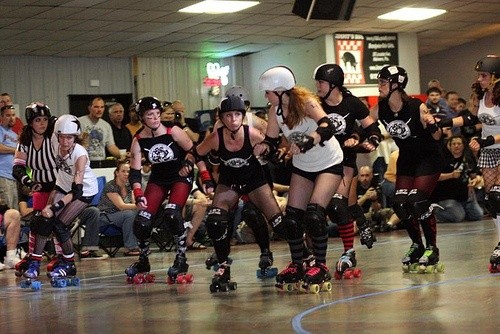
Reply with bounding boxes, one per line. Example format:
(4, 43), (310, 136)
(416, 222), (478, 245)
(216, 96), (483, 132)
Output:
(80, 250), (109, 260)
(4, 255), (21, 268)
(0, 262), (6, 271)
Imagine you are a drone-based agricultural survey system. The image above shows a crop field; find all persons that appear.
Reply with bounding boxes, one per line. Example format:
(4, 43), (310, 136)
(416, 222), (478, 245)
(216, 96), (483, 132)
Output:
(205, 86), (278, 277)
(344, 66), (446, 273)
(194, 97), (315, 302)
(313, 63), (381, 280)
(15, 102), (62, 280)
(423, 56), (500, 273)
(126, 96), (195, 284)
(207, 80), (500, 251)
(253, 66), (344, 293)
(0, 92), (212, 270)
(20, 114), (99, 290)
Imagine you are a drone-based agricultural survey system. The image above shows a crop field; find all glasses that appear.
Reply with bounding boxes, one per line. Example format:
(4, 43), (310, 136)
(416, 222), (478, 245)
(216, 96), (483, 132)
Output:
(378, 79), (389, 85)
(164, 102), (174, 107)
(1, 105), (14, 111)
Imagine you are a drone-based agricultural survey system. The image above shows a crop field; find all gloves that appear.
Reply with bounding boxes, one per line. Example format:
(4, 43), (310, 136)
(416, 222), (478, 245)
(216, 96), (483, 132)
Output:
(23, 177), (42, 191)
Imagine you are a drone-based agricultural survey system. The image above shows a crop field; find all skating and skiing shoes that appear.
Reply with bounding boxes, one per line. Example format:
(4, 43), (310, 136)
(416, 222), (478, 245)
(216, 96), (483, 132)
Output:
(125, 255), (155, 284)
(417, 244), (444, 273)
(14, 254), (79, 292)
(275, 220), (377, 292)
(257, 252), (278, 278)
(206, 253), (232, 269)
(489, 241), (500, 272)
(210, 261), (237, 293)
(167, 250), (194, 283)
(401, 242), (425, 273)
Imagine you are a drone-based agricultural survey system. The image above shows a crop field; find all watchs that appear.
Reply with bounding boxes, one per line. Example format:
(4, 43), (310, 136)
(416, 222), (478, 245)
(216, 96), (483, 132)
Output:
(369, 137), (380, 148)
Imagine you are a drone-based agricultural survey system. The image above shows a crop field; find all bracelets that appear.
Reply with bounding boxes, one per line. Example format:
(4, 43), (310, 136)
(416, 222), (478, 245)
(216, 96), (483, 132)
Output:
(437, 118), (453, 128)
(134, 188), (144, 200)
(349, 135), (358, 140)
(200, 170), (211, 181)
(50, 199), (64, 213)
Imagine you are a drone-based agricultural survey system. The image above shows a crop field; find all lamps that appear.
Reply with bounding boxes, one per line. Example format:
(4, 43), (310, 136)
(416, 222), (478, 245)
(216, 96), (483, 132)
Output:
(209, 84), (221, 96)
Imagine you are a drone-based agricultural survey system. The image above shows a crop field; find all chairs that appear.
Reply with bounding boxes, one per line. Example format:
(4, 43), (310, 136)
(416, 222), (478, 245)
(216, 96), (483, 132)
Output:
(91, 176), (124, 258)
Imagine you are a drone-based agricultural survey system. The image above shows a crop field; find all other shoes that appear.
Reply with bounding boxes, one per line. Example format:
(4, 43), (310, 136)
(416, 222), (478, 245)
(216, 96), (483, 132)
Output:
(183, 241), (206, 250)
(124, 248), (140, 256)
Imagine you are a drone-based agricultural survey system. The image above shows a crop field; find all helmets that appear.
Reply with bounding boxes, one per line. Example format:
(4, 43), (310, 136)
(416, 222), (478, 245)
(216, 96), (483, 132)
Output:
(135, 96), (163, 113)
(25, 102), (51, 123)
(257, 67), (295, 91)
(225, 86), (250, 106)
(377, 66), (408, 88)
(54, 114), (81, 135)
(313, 64), (344, 87)
(218, 96), (246, 115)
(473, 55), (500, 78)
